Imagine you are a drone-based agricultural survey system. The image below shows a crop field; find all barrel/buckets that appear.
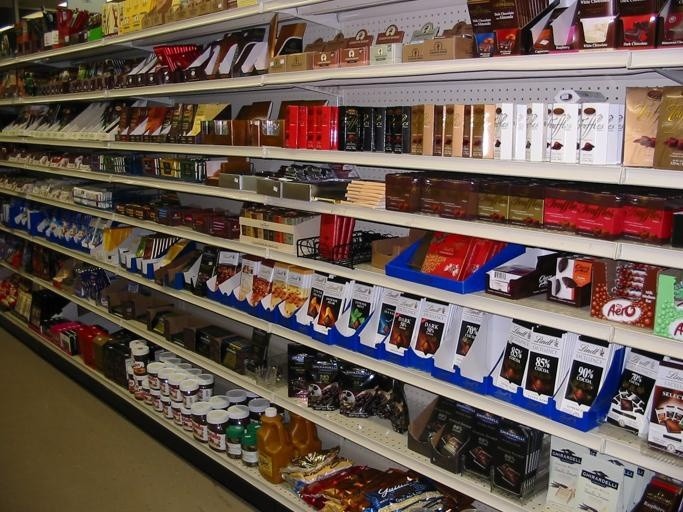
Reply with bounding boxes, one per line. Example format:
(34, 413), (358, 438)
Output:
(289, 417), (322, 458)
(256, 408), (293, 483)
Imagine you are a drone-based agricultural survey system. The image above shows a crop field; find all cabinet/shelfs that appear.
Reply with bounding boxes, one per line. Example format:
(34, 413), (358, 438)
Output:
(1, 2), (682, 510)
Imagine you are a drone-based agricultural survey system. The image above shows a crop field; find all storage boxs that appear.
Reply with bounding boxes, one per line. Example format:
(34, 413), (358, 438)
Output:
(406, 396), (550, 503)
(218, 172), (318, 202)
(73, 184), (113, 210)
(118, 1), (239, 35)
(268, 35), (475, 73)
(114, 100), (623, 166)
(385, 169), (673, 246)
(382, 232), (682, 345)
(183, 320), (253, 376)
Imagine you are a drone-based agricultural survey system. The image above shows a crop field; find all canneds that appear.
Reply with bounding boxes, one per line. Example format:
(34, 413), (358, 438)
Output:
(385, 171), (675, 245)
(125, 340), (290, 466)
